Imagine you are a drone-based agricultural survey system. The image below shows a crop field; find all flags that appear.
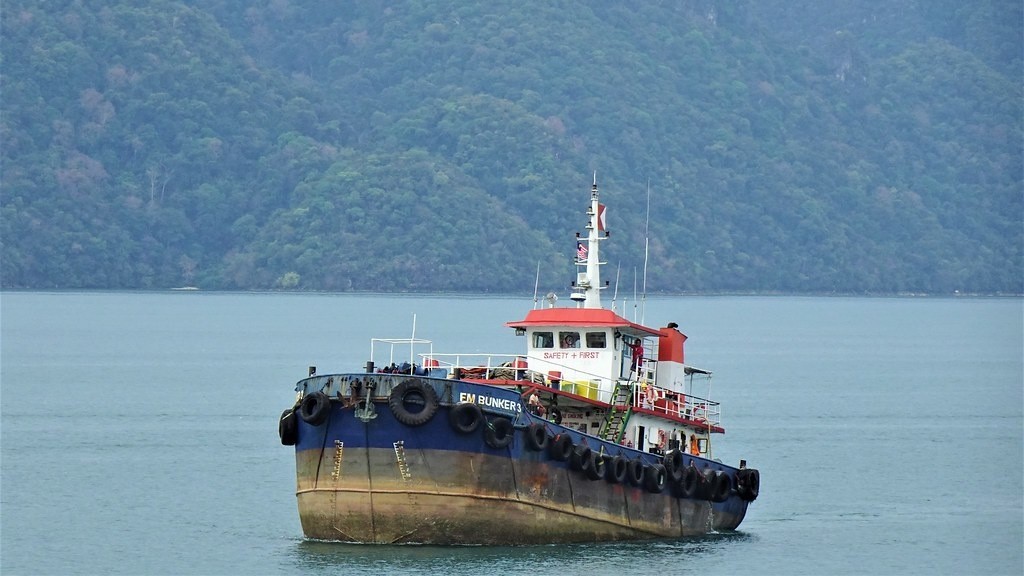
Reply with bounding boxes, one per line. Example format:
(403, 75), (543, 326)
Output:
(577, 242), (588, 260)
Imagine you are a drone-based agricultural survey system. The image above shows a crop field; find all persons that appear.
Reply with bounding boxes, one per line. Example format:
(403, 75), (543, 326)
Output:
(377, 363), (416, 375)
(622, 337), (643, 381)
(615, 431), (633, 447)
(515, 385), (563, 425)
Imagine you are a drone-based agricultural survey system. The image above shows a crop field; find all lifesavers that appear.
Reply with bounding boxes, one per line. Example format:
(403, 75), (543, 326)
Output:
(388, 378), (440, 426)
(550, 432), (575, 463)
(656, 430), (668, 449)
(645, 463), (668, 493)
(300, 391), (332, 427)
(712, 471), (732, 503)
(646, 385), (655, 405)
(698, 468), (719, 501)
(676, 465), (699, 498)
(450, 401), (484, 435)
(482, 417), (515, 450)
(607, 456), (627, 483)
(586, 453), (607, 481)
(278, 408), (298, 446)
(691, 405), (709, 424)
(628, 458), (645, 487)
(526, 422), (549, 451)
(741, 469), (759, 501)
(572, 445), (592, 472)
(663, 448), (684, 483)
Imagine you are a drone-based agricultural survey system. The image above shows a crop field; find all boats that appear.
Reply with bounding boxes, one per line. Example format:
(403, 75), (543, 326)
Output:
(278, 169), (760, 546)
